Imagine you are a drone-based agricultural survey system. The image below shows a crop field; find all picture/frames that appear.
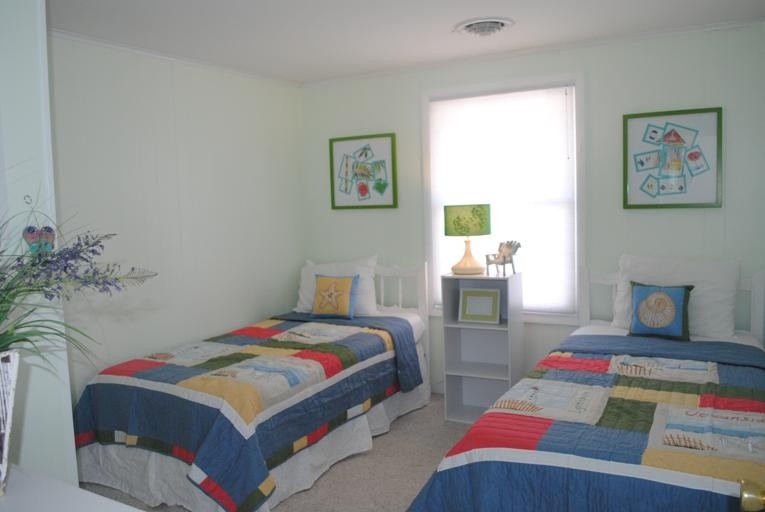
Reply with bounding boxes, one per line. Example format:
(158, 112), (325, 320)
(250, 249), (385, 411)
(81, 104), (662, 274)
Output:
(328, 131), (398, 209)
(457, 286), (501, 325)
(622, 104), (725, 210)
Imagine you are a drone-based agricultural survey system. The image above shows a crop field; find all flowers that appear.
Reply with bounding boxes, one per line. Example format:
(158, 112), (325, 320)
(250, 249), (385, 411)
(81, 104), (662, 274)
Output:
(0, 185), (160, 372)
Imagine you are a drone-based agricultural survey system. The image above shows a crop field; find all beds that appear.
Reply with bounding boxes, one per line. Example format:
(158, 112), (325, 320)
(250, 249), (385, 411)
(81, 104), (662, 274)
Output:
(464, 266), (765, 512)
(83, 260), (427, 511)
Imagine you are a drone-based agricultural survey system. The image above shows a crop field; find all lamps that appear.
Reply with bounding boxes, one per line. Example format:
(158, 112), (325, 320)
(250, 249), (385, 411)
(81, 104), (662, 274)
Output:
(444, 204), (491, 275)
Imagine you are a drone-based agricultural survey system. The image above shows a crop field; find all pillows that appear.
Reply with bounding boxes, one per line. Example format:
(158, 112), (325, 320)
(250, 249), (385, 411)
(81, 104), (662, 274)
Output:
(292, 255), (380, 319)
(611, 252), (742, 343)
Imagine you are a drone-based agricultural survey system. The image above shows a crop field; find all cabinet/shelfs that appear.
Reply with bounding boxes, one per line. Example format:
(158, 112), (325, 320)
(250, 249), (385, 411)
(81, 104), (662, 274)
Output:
(439, 268), (524, 424)
